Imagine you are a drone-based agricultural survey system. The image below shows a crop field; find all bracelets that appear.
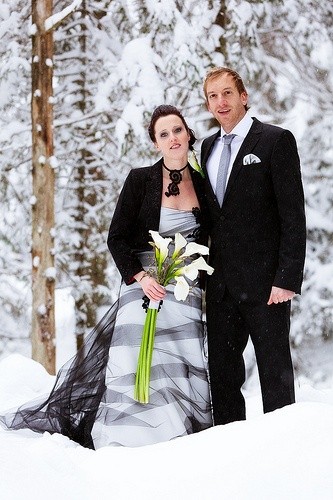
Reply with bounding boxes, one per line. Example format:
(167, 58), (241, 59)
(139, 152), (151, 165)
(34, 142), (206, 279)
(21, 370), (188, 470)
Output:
(138, 268), (156, 283)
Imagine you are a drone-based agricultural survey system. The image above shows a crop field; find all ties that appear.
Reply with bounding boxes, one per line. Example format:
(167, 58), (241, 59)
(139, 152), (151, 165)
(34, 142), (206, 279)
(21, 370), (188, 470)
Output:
(215, 134), (235, 208)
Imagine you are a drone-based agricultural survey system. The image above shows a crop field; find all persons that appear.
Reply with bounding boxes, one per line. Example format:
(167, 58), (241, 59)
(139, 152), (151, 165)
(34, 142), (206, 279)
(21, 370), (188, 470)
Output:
(199, 66), (310, 428)
(89, 103), (215, 449)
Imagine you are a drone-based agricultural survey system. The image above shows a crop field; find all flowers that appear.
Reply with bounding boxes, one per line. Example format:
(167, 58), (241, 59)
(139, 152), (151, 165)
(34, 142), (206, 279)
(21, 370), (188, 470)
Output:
(132, 229), (217, 405)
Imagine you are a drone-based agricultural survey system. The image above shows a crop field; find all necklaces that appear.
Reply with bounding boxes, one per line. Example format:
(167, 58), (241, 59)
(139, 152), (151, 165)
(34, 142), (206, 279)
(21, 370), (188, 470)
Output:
(162, 162), (190, 198)
